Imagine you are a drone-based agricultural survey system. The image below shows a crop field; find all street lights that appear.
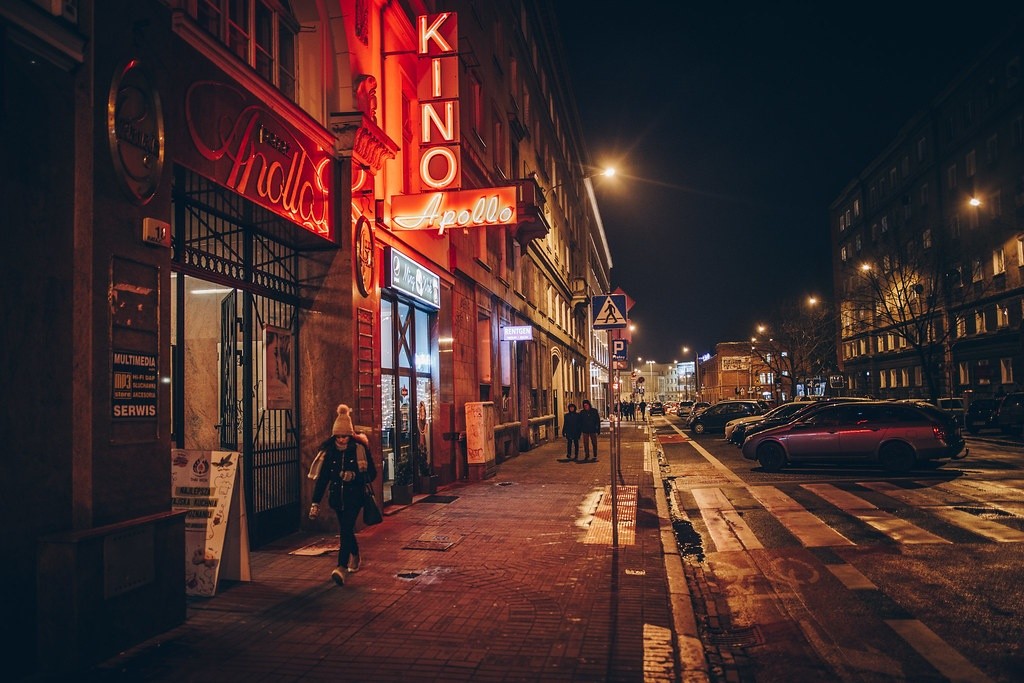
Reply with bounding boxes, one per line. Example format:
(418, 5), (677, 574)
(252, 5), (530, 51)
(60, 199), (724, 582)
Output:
(683, 346), (698, 392)
(645, 361), (655, 381)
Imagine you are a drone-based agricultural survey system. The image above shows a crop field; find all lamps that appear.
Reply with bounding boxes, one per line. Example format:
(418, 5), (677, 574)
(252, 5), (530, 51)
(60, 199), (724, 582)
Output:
(545, 167), (616, 199)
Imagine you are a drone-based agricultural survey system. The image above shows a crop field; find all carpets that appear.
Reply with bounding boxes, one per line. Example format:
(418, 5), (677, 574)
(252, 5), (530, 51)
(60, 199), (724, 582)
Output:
(416, 496), (459, 503)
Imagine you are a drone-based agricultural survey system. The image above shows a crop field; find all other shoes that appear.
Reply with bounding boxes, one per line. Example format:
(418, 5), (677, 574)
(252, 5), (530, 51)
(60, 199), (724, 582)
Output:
(332, 566), (348, 584)
(348, 554), (361, 572)
(574, 458), (578, 461)
(585, 455), (589, 460)
(593, 457), (597, 462)
(566, 454), (571, 458)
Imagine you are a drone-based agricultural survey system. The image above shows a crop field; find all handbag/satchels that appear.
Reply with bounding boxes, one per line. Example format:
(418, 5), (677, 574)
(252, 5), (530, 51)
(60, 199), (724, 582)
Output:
(363, 483), (382, 526)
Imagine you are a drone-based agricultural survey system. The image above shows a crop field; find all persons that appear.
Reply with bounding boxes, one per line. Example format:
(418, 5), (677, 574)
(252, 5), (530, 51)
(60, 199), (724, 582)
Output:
(562, 404), (581, 461)
(308, 405), (377, 584)
(608, 399), (647, 422)
(401, 401), (428, 459)
(579, 400), (601, 462)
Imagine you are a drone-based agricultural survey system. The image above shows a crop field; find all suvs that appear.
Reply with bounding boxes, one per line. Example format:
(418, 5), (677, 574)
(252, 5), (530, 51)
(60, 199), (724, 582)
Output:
(742, 398), (965, 475)
(997, 391), (1024, 435)
(729, 396), (872, 447)
(725, 400), (817, 439)
(935, 397), (967, 428)
(676, 400), (694, 416)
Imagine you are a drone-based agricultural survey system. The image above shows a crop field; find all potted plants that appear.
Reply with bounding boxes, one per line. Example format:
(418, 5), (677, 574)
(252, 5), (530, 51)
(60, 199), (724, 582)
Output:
(414, 444), (439, 495)
(390, 453), (414, 505)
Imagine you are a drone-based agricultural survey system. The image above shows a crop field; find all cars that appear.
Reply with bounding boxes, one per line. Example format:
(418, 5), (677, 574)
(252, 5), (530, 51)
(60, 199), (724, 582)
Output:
(963, 396), (1002, 436)
(650, 402), (666, 416)
(688, 402), (711, 415)
(663, 401), (679, 413)
(686, 398), (773, 435)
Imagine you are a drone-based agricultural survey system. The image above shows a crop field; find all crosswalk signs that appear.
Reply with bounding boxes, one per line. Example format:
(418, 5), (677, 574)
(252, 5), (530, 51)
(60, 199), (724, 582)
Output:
(590, 294), (627, 330)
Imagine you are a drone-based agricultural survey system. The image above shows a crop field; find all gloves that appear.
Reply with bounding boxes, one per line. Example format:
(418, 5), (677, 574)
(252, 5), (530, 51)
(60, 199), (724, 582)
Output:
(308, 503), (321, 520)
(342, 471), (356, 482)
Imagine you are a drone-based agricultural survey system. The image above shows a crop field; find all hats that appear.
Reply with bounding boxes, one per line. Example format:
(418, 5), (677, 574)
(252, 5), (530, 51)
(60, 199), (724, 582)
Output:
(331, 404), (355, 438)
